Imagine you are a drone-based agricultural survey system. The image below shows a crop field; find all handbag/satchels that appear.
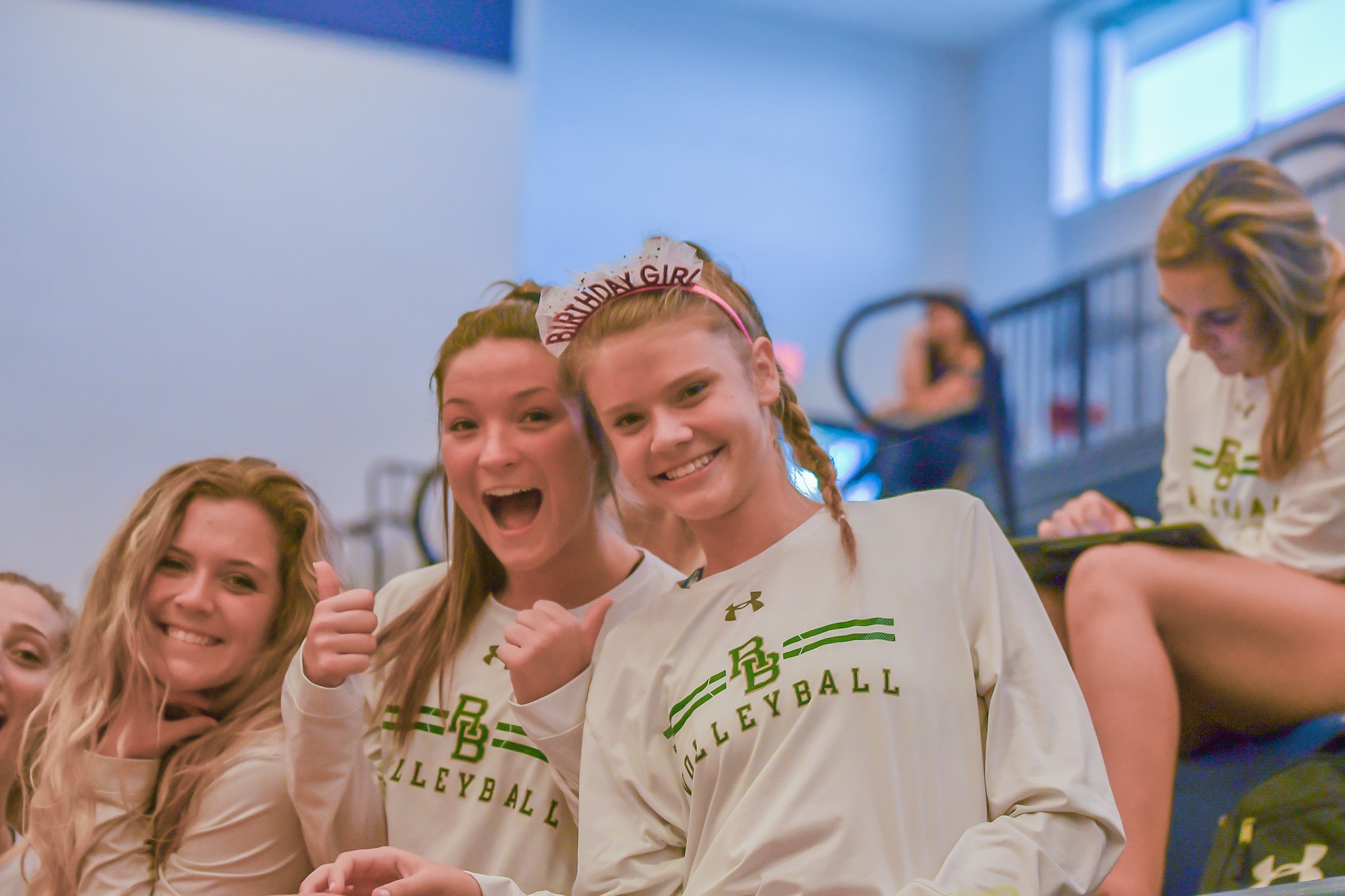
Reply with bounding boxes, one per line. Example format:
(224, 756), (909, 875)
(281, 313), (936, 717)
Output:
(1200, 733), (1344, 894)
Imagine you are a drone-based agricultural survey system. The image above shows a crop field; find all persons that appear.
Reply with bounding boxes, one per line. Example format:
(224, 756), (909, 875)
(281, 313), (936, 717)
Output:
(1023, 157), (1345, 896)
(280, 279), (687, 896)
(534, 235), (1126, 896)
(856, 294), (988, 493)
(29, 456), (313, 896)
(0, 573), (76, 896)
